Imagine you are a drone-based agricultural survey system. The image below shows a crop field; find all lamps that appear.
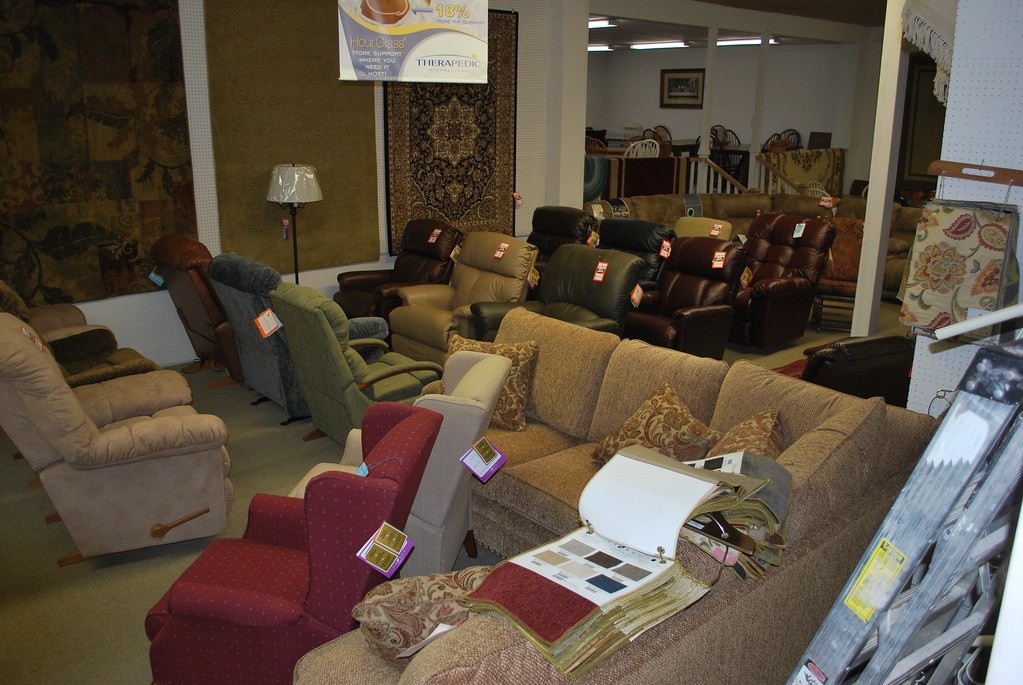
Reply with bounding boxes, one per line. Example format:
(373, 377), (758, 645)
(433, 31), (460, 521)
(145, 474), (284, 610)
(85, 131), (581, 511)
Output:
(628, 42), (693, 49)
(716, 37), (783, 46)
(587, 44), (615, 52)
(588, 19), (619, 29)
(266, 162), (323, 285)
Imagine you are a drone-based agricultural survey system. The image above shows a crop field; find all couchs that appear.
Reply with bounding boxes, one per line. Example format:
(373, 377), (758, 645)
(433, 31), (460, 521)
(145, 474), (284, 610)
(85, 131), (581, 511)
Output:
(0, 191), (925, 685)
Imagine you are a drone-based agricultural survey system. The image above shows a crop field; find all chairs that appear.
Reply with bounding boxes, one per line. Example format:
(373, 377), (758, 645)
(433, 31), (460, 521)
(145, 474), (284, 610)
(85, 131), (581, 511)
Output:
(585, 124), (832, 194)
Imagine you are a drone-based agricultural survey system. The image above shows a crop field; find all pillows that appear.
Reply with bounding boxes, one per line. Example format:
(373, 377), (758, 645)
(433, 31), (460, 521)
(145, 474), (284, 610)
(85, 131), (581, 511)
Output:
(350, 565), (494, 666)
(589, 381), (722, 465)
(439, 330), (539, 432)
(705, 408), (787, 462)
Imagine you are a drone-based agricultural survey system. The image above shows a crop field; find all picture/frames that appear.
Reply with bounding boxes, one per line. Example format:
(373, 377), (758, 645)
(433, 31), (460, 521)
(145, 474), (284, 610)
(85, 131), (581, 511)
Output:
(660, 68), (705, 109)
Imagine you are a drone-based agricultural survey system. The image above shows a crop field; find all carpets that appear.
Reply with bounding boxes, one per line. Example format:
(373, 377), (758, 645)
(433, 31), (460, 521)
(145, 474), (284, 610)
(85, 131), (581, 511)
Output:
(897, 200), (1020, 346)
(608, 158), (691, 200)
(203, 1), (381, 275)
(384, 10), (515, 256)
(756, 147), (845, 196)
(583, 155), (611, 203)
(0, 0), (198, 307)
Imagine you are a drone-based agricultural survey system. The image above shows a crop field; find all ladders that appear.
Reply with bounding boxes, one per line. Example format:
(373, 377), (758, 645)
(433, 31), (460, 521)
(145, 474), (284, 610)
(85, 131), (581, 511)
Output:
(786, 343), (1023, 685)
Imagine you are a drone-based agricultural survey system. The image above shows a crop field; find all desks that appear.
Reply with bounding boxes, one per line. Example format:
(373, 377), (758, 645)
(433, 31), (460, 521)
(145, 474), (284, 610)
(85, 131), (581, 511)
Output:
(662, 139), (700, 158)
(598, 137), (629, 156)
(713, 143), (760, 192)
